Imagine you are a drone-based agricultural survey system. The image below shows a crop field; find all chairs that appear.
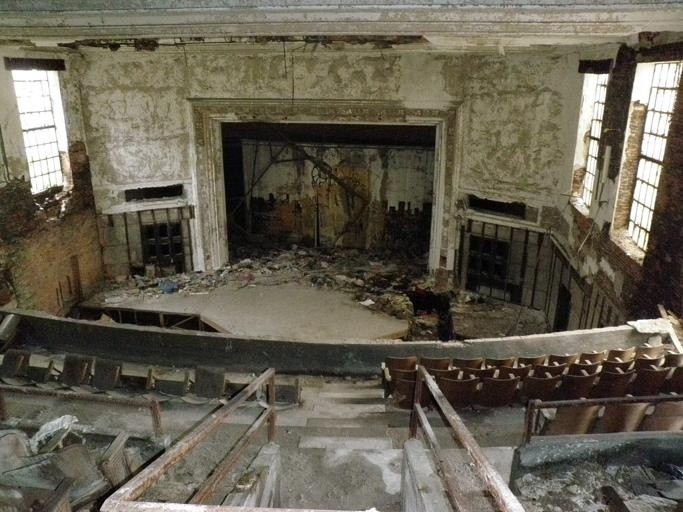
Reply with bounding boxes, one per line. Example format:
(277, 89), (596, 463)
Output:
(381, 342), (682, 409)
(0, 349), (300, 411)
(519, 392), (683, 437)
